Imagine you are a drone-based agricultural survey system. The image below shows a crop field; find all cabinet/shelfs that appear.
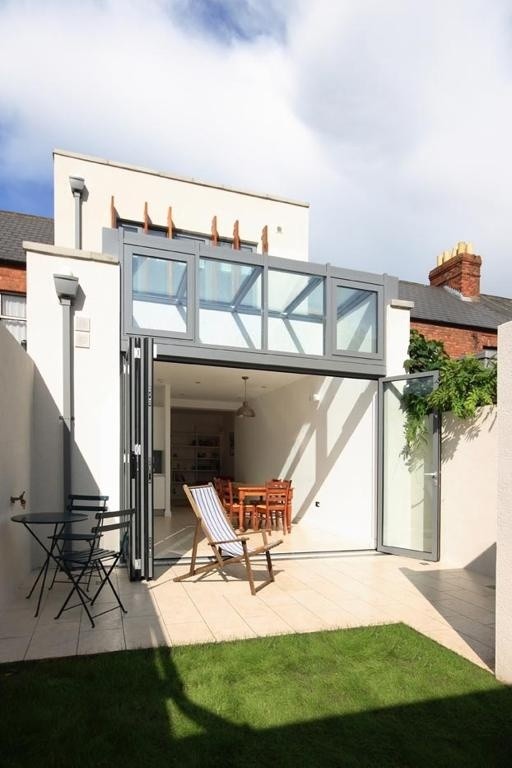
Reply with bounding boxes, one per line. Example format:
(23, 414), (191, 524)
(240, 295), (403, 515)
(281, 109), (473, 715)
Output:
(171, 423), (225, 506)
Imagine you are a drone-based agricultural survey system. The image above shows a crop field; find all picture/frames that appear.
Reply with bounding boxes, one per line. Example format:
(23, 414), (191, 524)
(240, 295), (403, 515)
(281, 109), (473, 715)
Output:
(229, 431), (234, 456)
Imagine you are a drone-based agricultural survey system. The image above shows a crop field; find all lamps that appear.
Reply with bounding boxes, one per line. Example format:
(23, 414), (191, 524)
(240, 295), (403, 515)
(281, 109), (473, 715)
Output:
(236, 376), (255, 418)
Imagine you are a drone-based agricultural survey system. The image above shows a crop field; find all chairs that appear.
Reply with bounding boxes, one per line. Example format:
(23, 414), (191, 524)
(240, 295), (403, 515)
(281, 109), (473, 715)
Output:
(54, 507), (137, 628)
(173, 477), (293, 595)
(47, 495), (110, 592)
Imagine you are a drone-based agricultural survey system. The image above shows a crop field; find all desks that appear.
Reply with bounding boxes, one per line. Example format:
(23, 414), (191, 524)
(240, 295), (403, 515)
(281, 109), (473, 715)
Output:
(11, 512), (93, 618)
